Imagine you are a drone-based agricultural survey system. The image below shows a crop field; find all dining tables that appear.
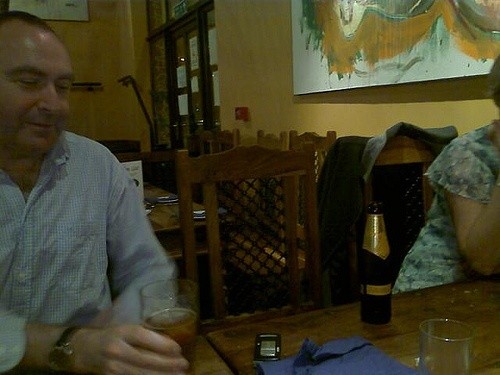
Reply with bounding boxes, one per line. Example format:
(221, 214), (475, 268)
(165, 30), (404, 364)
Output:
(177, 280), (500, 375)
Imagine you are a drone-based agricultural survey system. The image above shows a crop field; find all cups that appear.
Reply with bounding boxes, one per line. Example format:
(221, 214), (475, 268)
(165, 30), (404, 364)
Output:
(419, 319), (476, 375)
(139, 279), (200, 372)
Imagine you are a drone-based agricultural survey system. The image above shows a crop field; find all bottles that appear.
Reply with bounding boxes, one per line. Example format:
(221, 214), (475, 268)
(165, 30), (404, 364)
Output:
(360, 200), (391, 324)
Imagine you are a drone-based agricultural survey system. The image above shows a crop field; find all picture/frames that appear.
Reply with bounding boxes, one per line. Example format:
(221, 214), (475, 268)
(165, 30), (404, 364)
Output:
(9, 0), (90, 22)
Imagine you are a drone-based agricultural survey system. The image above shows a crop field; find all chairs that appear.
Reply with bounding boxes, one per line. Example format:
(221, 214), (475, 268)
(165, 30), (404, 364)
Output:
(178, 121), (459, 335)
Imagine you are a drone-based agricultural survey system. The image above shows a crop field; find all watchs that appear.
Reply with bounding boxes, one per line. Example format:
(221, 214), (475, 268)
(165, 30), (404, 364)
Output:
(47, 324), (82, 375)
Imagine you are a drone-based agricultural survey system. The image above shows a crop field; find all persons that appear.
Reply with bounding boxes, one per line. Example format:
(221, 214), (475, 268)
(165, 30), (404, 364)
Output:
(391, 54), (500, 295)
(0, 10), (193, 375)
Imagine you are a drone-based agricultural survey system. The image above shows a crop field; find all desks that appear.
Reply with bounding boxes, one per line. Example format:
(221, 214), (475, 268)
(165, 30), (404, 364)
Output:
(143, 182), (225, 261)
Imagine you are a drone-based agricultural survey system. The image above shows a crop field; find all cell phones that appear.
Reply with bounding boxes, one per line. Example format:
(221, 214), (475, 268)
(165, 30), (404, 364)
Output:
(253, 333), (281, 368)
